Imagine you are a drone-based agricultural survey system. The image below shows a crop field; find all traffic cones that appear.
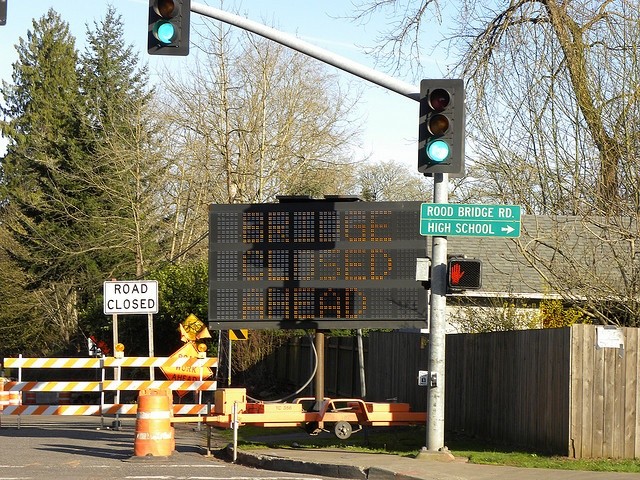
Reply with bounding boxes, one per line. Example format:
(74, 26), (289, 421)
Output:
(0, 375), (9, 412)
(25, 388), (36, 405)
(7, 380), (22, 406)
(122, 392), (179, 463)
(57, 391), (69, 404)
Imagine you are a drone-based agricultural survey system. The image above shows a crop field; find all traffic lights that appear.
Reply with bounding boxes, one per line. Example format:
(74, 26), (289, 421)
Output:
(448, 259), (482, 289)
(418, 79), (465, 174)
(147, 0), (190, 56)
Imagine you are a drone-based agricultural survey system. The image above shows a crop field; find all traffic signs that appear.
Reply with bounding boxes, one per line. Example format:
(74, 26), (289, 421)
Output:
(103, 280), (158, 315)
(159, 341), (213, 398)
(420, 202), (521, 239)
